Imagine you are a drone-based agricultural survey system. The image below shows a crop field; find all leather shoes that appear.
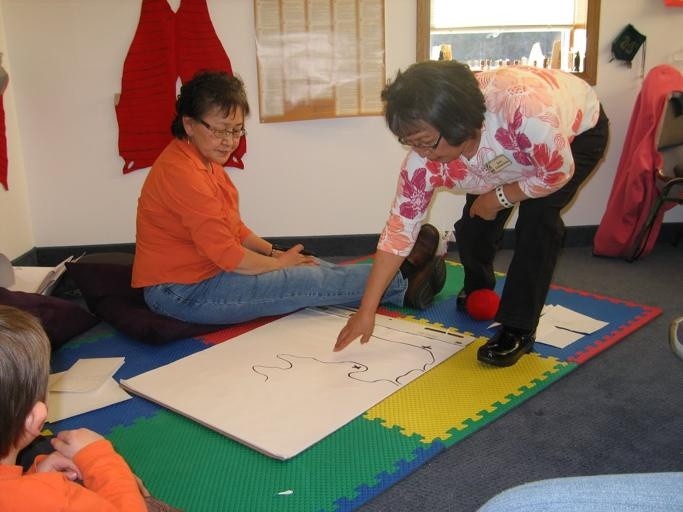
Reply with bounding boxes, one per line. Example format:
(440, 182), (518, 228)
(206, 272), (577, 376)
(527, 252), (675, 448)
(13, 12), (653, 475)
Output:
(476, 320), (535, 368)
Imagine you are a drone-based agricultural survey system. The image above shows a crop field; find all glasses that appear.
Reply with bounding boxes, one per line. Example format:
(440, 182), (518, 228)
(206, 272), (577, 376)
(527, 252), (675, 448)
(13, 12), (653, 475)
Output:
(397, 129), (445, 154)
(196, 115), (247, 139)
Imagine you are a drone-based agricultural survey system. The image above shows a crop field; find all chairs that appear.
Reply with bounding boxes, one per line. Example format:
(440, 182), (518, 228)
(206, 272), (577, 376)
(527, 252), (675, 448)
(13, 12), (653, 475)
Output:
(625, 64), (683, 263)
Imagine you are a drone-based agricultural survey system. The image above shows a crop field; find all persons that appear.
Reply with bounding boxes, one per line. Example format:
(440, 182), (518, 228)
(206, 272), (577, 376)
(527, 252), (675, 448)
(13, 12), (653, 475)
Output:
(330, 57), (610, 370)
(0, 302), (149, 510)
(128, 66), (448, 326)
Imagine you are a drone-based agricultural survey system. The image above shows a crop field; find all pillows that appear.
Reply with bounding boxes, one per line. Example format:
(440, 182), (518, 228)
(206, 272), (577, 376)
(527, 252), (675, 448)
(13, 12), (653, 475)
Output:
(52, 252), (137, 298)
(0, 286), (101, 353)
(65, 262), (238, 347)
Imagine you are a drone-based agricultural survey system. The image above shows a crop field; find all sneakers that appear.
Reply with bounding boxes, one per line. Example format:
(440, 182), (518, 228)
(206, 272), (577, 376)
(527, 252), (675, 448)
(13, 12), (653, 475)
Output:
(405, 255), (447, 310)
(456, 284), (496, 313)
(406, 223), (440, 271)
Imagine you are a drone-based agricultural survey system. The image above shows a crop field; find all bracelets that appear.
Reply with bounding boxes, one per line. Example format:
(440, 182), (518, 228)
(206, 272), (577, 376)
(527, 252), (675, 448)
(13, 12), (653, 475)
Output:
(267, 243), (279, 256)
(494, 185), (515, 210)
